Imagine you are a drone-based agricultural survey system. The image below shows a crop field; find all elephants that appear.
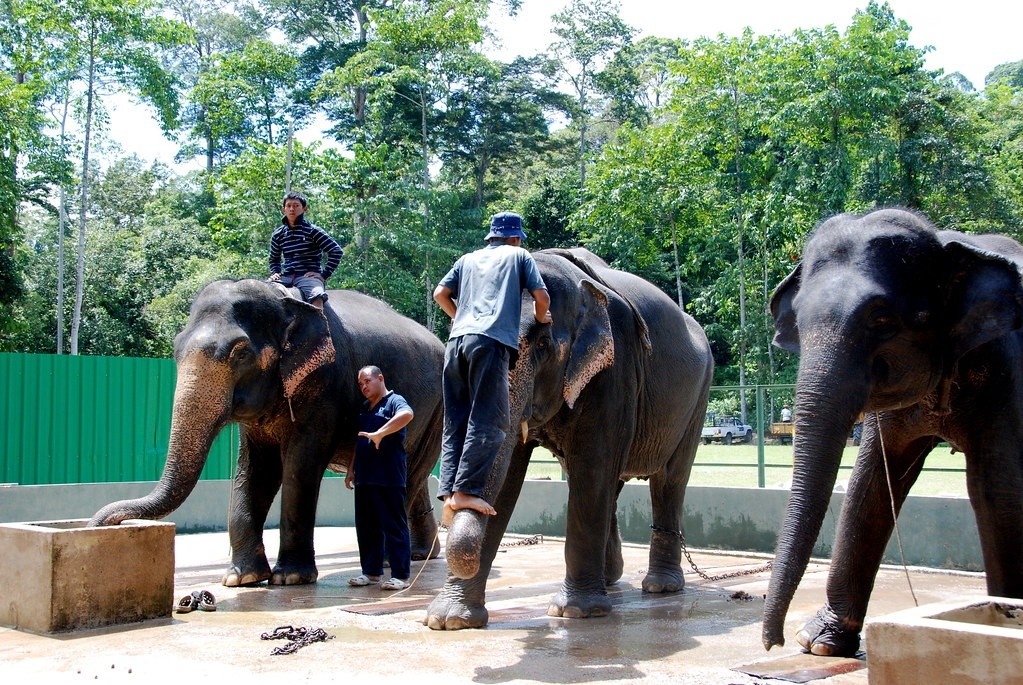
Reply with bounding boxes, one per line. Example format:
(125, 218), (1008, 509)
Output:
(419, 245), (716, 632)
(85, 276), (442, 591)
(759, 205), (1021, 659)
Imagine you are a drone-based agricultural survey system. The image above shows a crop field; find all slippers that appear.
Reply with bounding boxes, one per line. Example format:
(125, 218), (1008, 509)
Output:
(379, 578), (410, 589)
(176, 595), (198, 613)
(192, 589), (216, 611)
(347, 575), (377, 586)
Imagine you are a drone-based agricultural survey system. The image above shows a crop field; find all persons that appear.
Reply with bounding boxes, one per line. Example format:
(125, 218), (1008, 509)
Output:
(264, 190), (343, 316)
(431, 209), (554, 528)
(344, 366), (413, 590)
(779, 404), (793, 423)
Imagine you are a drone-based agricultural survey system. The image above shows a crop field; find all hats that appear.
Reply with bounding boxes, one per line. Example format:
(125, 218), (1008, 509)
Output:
(484, 212), (527, 240)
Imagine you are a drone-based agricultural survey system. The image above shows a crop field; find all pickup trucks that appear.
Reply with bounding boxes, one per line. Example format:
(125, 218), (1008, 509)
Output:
(700, 414), (753, 445)
